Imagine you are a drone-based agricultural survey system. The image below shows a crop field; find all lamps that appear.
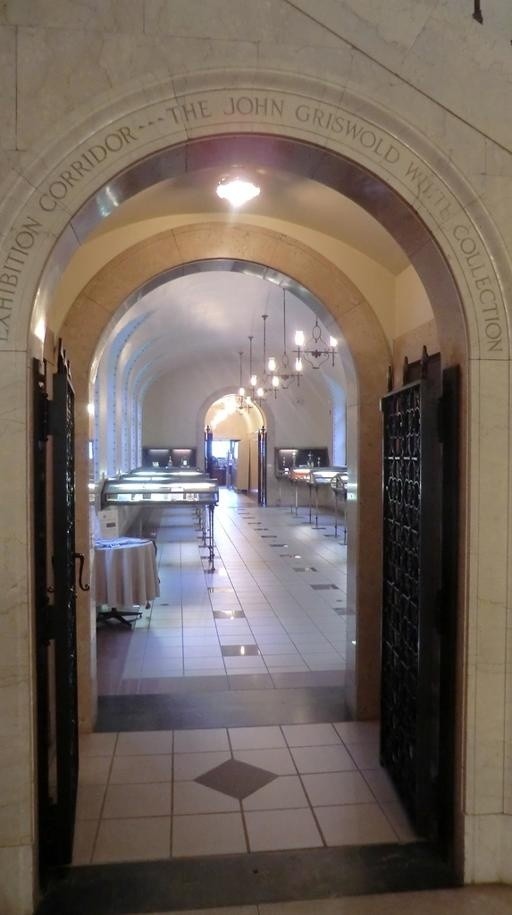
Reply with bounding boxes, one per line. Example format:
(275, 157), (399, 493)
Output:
(214, 164), (260, 207)
(238, 287), (337, 414)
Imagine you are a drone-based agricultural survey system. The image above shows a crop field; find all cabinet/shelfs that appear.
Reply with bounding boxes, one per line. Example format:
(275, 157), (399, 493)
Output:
(100, 466), (219, 573)
(274, 446), (347, 546)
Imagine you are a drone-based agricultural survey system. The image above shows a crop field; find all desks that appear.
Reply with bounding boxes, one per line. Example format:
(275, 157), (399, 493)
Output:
(95, 537), (159, 629)
